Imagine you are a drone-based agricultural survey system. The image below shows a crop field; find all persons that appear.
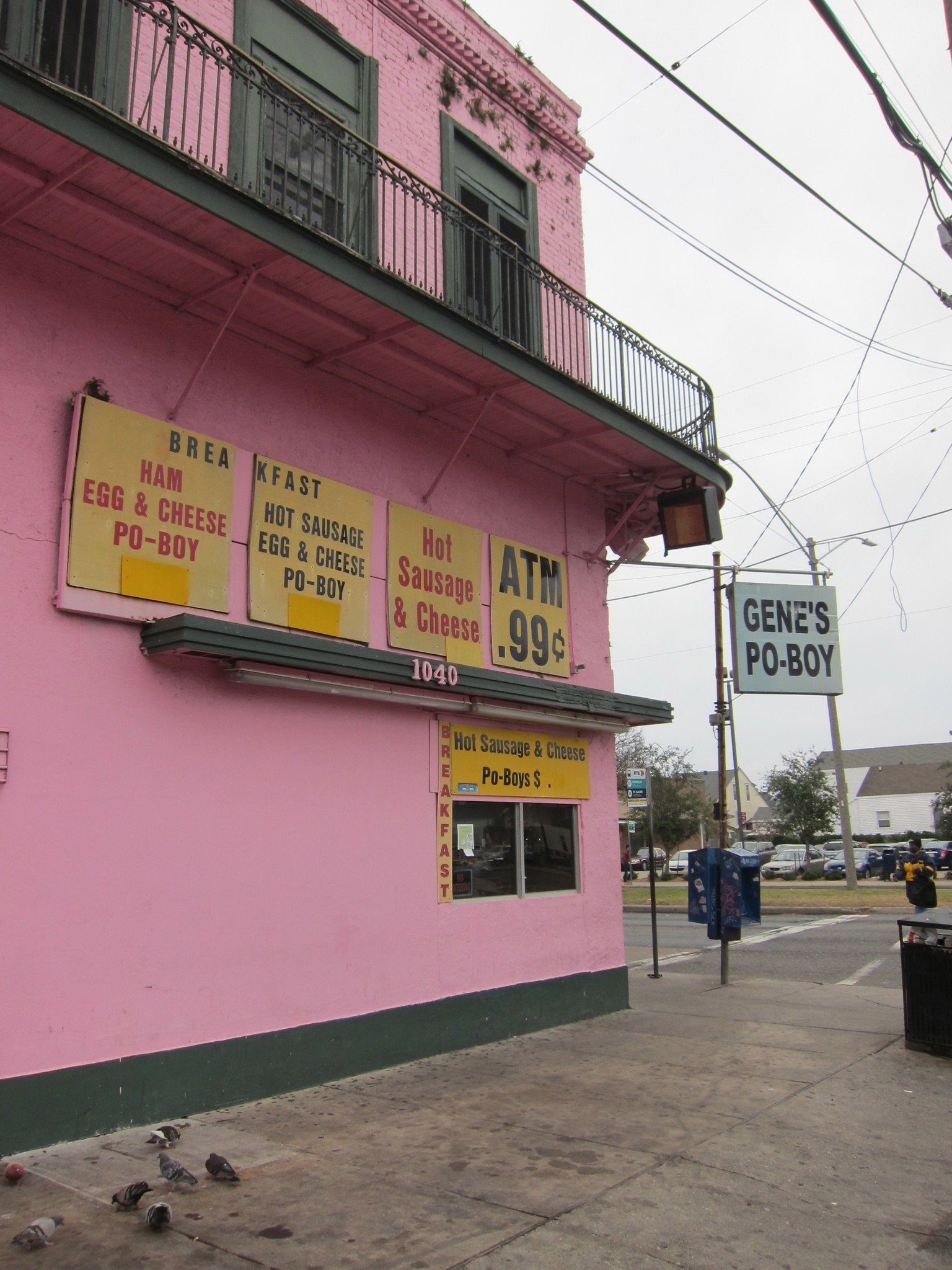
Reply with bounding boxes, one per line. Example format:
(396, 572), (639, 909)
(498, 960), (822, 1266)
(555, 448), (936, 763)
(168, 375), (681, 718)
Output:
(893, 837), (937, 943)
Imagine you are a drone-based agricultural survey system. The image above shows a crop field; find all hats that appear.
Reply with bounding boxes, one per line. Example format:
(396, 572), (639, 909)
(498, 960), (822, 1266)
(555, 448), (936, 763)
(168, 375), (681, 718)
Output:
(909, 838), (922, 847)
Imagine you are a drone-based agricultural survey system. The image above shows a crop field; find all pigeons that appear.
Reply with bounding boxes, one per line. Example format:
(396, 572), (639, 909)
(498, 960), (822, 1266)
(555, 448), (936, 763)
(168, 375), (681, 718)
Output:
(145, 1126), (185, 1150)
(146, 1203), (173, 1234)
(14, 1215), (64, 1248)
(3, 1161), (24, 1188)
(111, 1180), (151, 1210)
(204, 1153), (237, 1177)
(156, 1150), (198, 1190)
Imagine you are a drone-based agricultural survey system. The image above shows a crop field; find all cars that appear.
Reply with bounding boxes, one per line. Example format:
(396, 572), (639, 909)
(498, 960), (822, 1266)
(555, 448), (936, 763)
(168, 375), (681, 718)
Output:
(621, 838), (952, 881)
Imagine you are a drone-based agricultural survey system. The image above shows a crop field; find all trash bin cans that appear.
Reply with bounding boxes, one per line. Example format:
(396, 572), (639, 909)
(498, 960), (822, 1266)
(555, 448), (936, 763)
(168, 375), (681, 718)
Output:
(896, 908), (952, 1058)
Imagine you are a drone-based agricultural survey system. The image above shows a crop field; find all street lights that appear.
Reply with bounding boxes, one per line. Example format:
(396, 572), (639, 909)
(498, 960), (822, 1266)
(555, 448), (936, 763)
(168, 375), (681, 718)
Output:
(707, 444), (878, 888)
